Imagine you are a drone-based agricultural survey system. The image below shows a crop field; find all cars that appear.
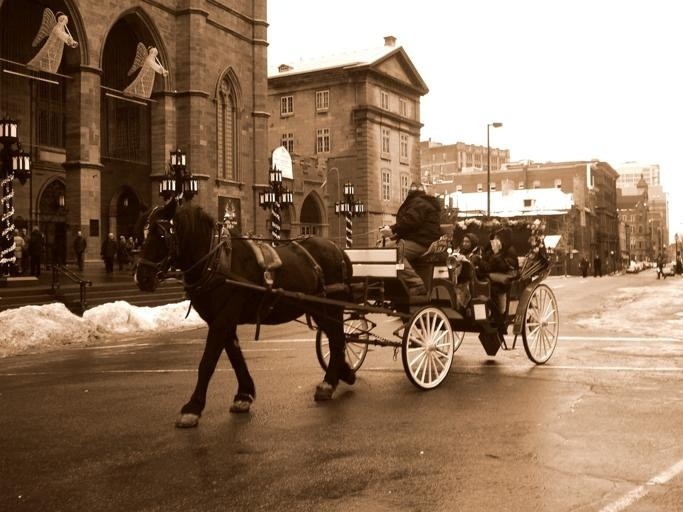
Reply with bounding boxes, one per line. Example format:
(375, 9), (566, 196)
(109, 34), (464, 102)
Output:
(627, 261), (651, 273)
(663, 262), (675, 276)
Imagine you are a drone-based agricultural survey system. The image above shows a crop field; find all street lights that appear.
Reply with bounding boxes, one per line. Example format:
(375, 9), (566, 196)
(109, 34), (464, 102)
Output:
(335, 181), (363, 248)
(0, 115), (32, 278)
(260, 166), (292, 246)
(487, 122), (504, 219)
(159, 147), (198, 271)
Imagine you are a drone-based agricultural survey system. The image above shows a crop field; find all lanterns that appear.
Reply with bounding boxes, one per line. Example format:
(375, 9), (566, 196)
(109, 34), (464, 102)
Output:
(59, 195), (65, 207)
(123, 197), (128, 208)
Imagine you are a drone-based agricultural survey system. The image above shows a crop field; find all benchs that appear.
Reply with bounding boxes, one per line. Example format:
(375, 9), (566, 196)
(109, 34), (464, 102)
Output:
(447, 226), (531, 299)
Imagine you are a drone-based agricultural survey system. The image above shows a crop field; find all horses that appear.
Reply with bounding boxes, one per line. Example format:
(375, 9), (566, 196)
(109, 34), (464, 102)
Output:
(134, 192), (357, 428)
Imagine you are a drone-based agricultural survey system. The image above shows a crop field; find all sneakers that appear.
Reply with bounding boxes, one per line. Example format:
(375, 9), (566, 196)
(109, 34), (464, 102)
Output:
(410, 285), (428, 297)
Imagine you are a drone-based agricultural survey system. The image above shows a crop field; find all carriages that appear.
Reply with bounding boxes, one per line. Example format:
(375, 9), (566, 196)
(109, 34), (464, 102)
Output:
(136, 196), (560, 430)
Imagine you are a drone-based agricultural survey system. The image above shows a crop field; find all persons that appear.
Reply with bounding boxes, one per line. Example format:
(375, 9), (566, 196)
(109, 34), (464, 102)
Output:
(13, 227), (141, 278)
(379, 182), (447, 296)
(123, 48), (168, 99)
(453, 233), (517, 282)
(673, 251), (682, 276)
(657, 253), (666, 279)
(594, 255), (602, 277)
(27, 14), (78, 74)
(581, 255), (589, 277)
